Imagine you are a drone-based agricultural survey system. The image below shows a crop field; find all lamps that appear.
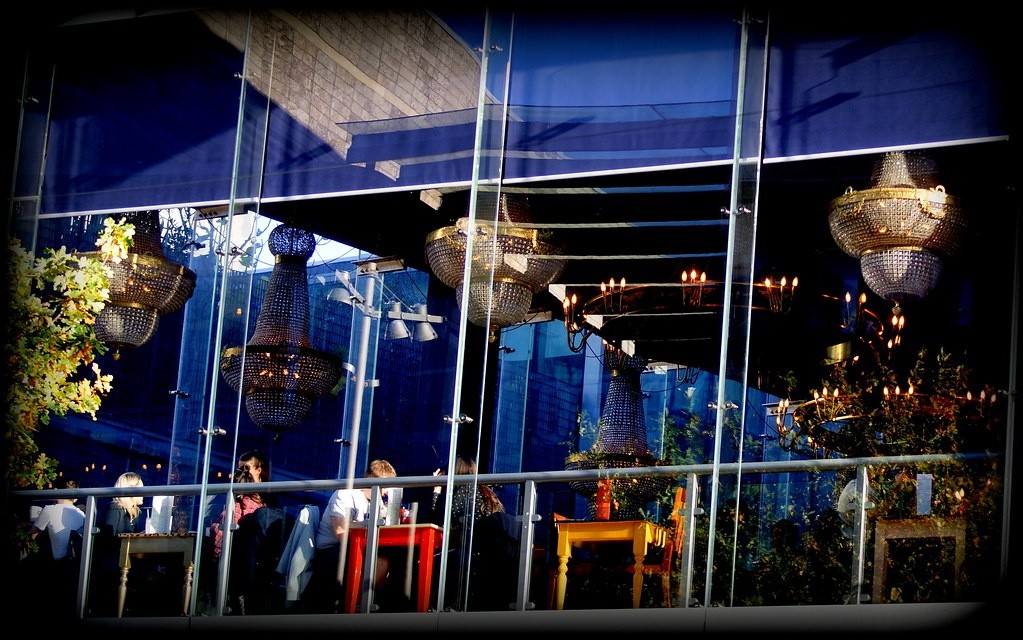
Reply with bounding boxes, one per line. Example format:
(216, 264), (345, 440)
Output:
(220, 222), (343, 440)
(563, 347), (668, 520)
(326, 270), (352, 309)
(62, 210), (198, 360)
(425, 191), (571, 344)
(828, 151), (972, 319)
(562, 267), (906, 371)
(383, 302), (413, 342)
(411, 303), (437, 342)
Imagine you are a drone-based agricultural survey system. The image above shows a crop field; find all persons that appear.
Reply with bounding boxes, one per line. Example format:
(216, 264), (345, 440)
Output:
(835, 448), (908, 602)
(211, 450), (281, 614)
(29, 479), (86, 580)
(418, 457), (505, 611)
(315, 460), (403, 612)
(93, 473), (145, 615)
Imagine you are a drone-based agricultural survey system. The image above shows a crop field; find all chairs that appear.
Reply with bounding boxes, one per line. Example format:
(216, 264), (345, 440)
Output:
(48, 529), (82, 618)
(626, 486), (685, 608)
(255, 502), (320, 614)
(446, 512), (522, 611)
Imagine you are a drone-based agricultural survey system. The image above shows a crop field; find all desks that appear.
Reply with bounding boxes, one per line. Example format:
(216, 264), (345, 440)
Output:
(345, 524), (443, 612)
(116, 530), (197, 618)
(871, 516), (967, 603)
(555, 523), (673, 610)
